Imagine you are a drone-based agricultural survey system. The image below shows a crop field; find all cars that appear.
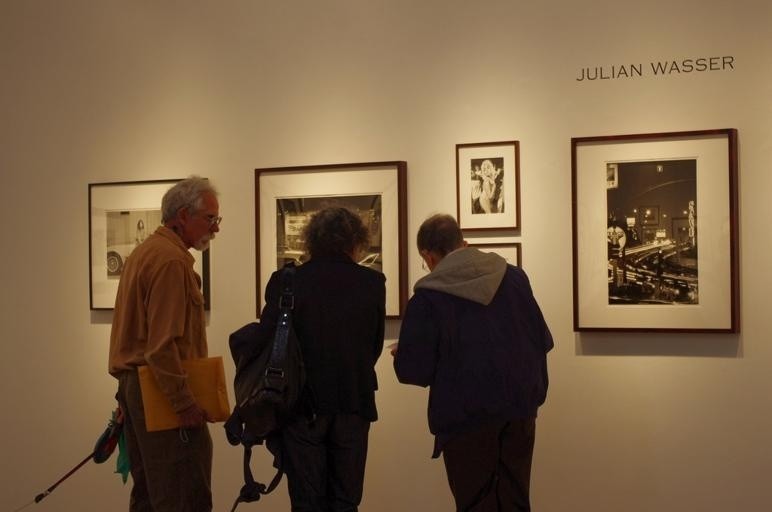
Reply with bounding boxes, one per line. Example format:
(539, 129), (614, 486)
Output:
(106, 242), (140, 276)
(635, 274), (693, 292)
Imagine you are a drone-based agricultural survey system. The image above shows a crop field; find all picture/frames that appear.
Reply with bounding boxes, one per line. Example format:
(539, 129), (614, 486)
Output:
(570, 128), (741, 334)
(456, 140), (521, 267)
(89, 176), (211, 312)
(253, 161), (408, 319)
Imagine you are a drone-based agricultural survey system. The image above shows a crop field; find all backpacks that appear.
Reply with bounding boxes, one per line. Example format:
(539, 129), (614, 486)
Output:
(222, 261), (305, 448)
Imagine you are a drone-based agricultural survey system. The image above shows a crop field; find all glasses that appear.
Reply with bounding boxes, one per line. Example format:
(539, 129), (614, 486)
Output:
(421, 257), (430, 272)
(197, 210), (222, 228)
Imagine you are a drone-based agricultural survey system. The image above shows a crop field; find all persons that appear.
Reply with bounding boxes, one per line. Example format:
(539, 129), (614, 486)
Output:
(107, 175), (222, 512)
(136, 218), (146, 245)
(390, 212), (554, 511)
(260, 206), (389, 512)
(473, 159), (503, 213)
